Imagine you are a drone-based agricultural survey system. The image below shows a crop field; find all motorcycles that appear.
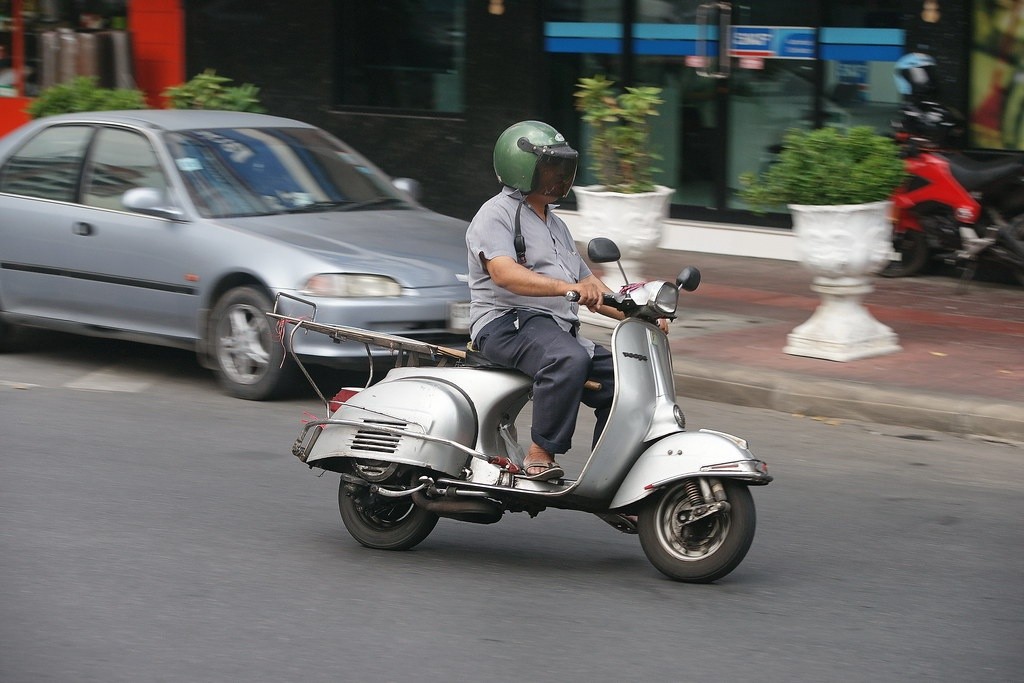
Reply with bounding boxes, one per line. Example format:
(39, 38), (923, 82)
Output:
(884, 66), (1021, 280)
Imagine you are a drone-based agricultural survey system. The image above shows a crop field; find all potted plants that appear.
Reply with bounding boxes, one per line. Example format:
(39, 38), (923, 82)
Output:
(732, 124), (916, 361)
(570, 74), (678, 293)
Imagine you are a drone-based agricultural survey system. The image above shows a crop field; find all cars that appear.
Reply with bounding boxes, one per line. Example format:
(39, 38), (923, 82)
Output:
(0, 108), (469, 399)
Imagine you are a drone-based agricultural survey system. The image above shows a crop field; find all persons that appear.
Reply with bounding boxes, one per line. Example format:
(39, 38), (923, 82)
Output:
(466, 120), (670, 481)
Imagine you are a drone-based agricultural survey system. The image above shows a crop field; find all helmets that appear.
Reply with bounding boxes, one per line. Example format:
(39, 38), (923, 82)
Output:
(491, 119), (579, 198)
(891, 50), (938, 98)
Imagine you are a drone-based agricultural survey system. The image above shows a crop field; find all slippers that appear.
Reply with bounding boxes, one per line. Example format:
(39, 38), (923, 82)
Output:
(522, 461), (563, 480)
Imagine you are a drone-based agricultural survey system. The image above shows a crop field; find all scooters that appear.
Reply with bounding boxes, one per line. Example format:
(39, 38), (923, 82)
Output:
(265, 237), (775, 581)
